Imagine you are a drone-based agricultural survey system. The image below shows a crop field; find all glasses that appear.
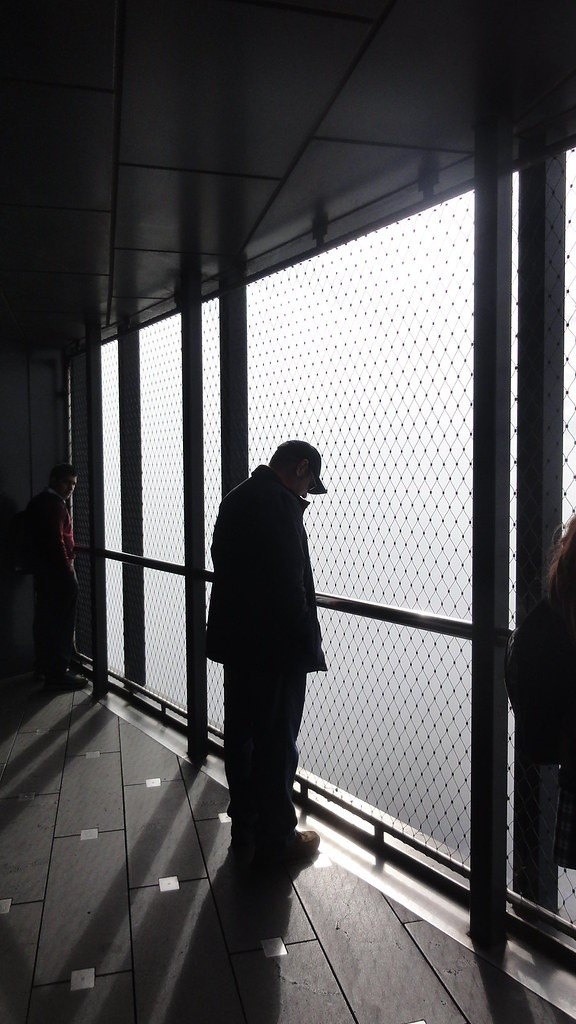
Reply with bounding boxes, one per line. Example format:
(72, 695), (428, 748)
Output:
(310, 472), (317, 490)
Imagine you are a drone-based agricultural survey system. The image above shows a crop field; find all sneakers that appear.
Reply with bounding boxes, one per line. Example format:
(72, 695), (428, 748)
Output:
(230, 831), (320, 862)
(33, 671), (88, 692)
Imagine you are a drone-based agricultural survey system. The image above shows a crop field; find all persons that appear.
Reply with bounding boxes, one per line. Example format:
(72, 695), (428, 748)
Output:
(505, 516), (576, 871)
(26, 463), (88, 691)
(204, 441), (327, 864)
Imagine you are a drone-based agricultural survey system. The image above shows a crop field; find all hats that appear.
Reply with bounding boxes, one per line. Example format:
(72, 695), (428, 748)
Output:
(277, 440), (327, 494)
